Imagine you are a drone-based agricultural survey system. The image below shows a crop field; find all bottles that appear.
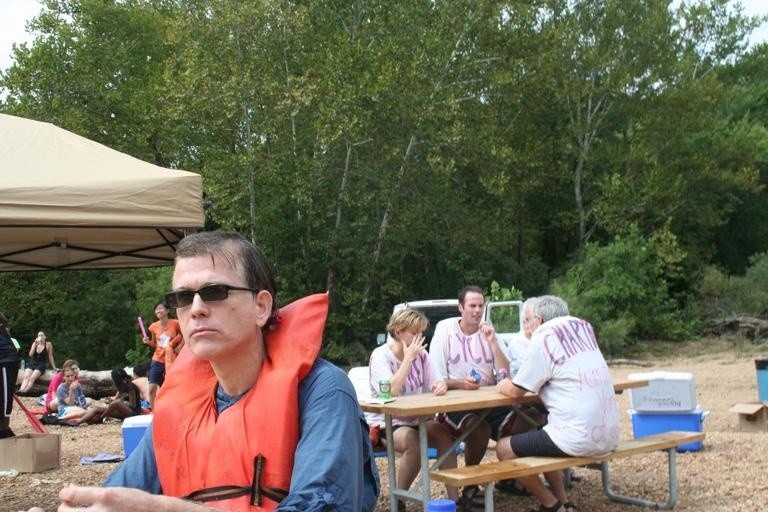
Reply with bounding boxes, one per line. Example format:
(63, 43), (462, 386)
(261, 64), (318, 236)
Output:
(508, 354), (522, 378)
(425, 499), (456, 512)
(469, 368), (481, 383)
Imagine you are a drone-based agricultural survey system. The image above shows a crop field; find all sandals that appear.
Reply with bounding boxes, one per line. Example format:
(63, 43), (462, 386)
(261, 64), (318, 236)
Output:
(564, 502), (579, 512)
(539, 501), (566, 511)
(461, 486), (480, 499)
(496, 480), (530, 496)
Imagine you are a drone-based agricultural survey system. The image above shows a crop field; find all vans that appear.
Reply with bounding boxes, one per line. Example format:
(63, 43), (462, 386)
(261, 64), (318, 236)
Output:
(375, 298), (527, 358)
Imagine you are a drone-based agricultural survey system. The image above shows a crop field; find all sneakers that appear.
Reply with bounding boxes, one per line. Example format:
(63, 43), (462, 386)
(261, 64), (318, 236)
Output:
(397, 500), (406, 511)
(15, 388), (32, 397)
(456, 497), (469, 512)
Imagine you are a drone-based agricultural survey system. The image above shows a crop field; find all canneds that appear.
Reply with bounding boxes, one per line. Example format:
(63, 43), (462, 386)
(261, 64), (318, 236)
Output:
(379, 379), (391, 399)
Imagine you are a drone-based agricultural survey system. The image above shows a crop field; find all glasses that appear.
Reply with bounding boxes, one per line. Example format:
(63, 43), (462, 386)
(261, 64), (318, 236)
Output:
(165, 284), (258, 309)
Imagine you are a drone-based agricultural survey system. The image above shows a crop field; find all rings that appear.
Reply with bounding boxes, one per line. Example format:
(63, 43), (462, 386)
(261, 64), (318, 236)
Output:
(438, 383), (442, 388)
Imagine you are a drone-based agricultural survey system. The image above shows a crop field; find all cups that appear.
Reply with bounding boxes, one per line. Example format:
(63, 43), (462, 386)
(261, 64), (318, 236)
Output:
(37, 336), (42, 343)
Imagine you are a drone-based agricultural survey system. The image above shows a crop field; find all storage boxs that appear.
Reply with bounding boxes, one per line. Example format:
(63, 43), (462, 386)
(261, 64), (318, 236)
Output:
(120, 411), (158, 463)
(0, 430), (64, 477)
(730, 355), (768, 433)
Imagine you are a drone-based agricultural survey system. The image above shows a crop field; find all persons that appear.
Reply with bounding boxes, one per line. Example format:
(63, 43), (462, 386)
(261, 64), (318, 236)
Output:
(14, 226), (383, 512)
(505, 295), (581, 491)
(365, 306), (487, 511)
(0, 299), (183, 437)
(494, 292), (621, 511)
(427, 282), (534, 508)
